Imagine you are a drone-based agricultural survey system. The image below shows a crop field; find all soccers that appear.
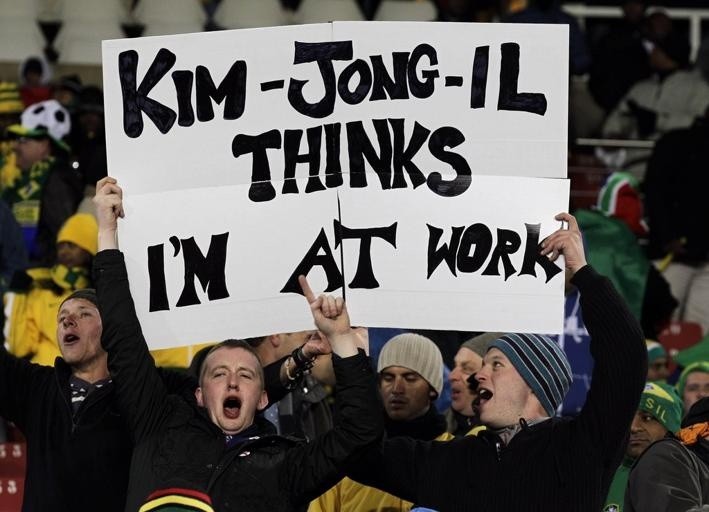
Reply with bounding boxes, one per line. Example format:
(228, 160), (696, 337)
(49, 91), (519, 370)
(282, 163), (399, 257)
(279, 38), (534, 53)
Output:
(21, 100), (72, 140)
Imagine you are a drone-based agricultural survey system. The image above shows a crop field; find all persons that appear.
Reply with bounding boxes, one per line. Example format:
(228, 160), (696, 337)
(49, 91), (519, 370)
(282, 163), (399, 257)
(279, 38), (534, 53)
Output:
(2, 0), (708, 511)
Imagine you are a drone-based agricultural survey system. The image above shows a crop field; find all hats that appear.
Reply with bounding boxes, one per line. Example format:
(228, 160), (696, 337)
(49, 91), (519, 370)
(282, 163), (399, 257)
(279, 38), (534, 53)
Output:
(72, 86), (103, 114)
(460, 333), (508, 359)
(6, 100), (72, 155)
(56, 213), (99, 257)
(58, 289), (105, 329)
(377, 333), (444, 401)
(486, 333), (573, 417)
(645, 338), (667, 362)
(49, 75), (83, 95)
(137, 479), (214, 512)
(678, 361), (708, 400)
(638, 381), (685, 435)
